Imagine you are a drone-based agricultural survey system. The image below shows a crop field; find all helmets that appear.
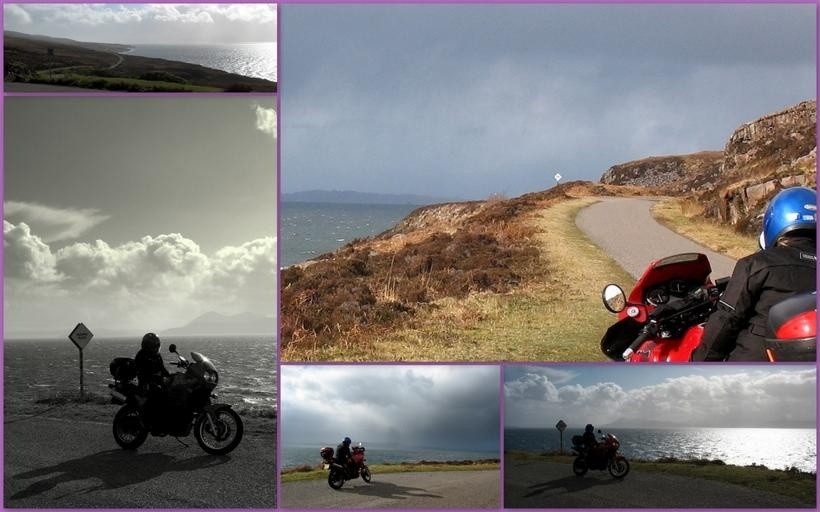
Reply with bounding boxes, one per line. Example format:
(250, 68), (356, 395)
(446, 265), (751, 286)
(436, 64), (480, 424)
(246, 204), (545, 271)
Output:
(762, 185), (817, 250)
(345, 437), (351, 444)
(585, 424), (593, 433)
(141, 333), (161, 353)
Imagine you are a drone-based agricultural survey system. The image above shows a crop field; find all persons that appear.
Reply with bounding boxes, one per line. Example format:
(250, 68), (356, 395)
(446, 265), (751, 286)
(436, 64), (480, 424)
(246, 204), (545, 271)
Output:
(335, 436), (357, 476)
(135, 332), (171, 438)
(582, 423), (599, 466)
(690, 185), (818, 363)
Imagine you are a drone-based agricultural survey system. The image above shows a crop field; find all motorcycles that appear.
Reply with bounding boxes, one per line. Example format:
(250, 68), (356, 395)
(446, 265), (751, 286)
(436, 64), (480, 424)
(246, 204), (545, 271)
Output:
(109, 344), (243, 454)
(601, 253), (816, 361)
(571, 430), (629, 478)
(320, 447), (371, 489)
